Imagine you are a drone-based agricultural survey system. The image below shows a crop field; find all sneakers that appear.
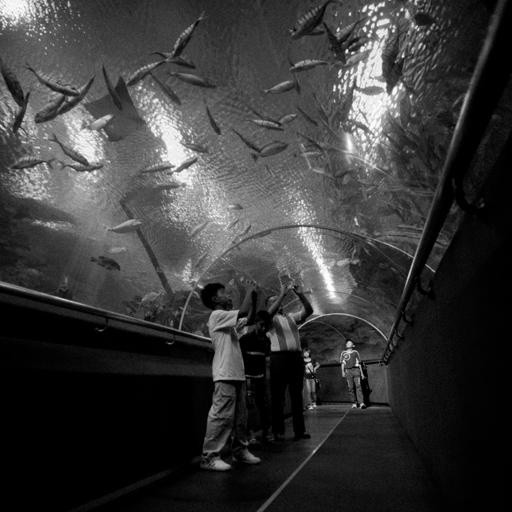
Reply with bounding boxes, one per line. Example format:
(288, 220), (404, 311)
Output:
(232, 451), (262, 465)
(352, 404), (357, 408)
(309, 403), (316, 409)
(200, 459), (231, 471)
(360, 403), (365, 408)
(295, 434), (310, 440)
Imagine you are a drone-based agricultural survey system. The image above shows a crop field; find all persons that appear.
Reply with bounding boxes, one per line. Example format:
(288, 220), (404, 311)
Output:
(302, 347), (321, 410)
(200, 282), (263, 471)
(339, 339), (368, 408)
(361, 361), (372, 406)
(241, 310), (274, 453)
(264, 275), (313, 442)
(313, 364), (322, 405)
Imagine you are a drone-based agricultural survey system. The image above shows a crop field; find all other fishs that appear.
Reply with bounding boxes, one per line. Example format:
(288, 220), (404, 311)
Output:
(290, 0), (493, 223)
